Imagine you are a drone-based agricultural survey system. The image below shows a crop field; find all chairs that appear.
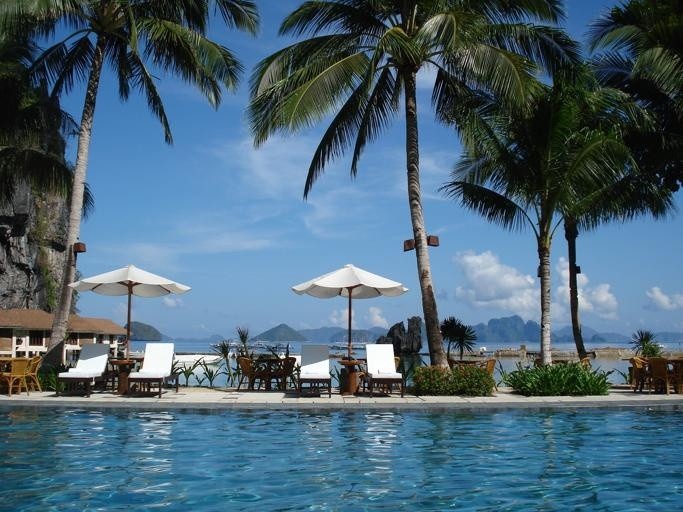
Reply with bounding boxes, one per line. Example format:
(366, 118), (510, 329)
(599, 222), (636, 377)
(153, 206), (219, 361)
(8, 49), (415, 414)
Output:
(630, 355), (683, 395)
(236, 344), (404, 398)
(0, 356), (43, 396)
(533, 357), (592, 373)
(128, 343), (179, 398)
(447, 358), (499, 391)
(56, 343), (110, 397)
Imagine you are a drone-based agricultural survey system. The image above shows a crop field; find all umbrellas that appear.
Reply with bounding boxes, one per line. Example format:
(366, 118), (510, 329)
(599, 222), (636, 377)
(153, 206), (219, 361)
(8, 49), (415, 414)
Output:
(291, 263), (410, 361)
(67, 263), (193, 358)
